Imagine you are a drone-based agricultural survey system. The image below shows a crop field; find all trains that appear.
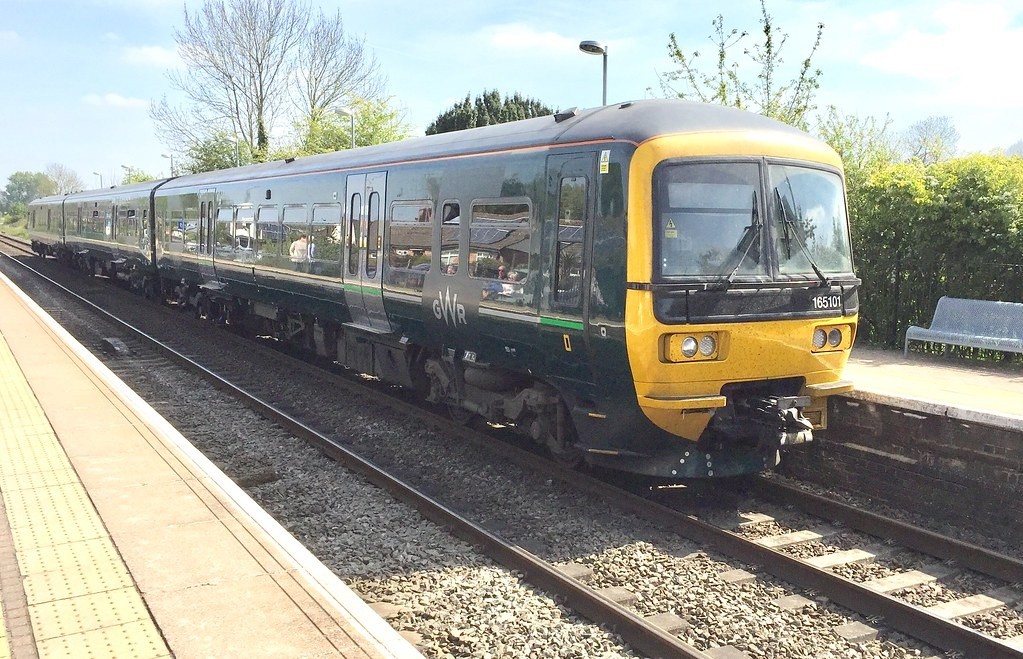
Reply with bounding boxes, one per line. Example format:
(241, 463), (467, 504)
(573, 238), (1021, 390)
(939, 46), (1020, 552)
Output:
(23, 101), (863, 479)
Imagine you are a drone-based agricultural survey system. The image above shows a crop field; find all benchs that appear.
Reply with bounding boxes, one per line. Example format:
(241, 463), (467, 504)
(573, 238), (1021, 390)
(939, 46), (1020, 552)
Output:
(904, 296), (1023, 358)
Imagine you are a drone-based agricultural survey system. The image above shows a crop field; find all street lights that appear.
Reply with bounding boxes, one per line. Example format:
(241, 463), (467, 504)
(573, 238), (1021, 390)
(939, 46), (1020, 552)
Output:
(334, 107), (356, 149)
(580, 41), (608, 104)
(222, 137), (239, 167)
(93, 171), (102, 189)
(121, 165), (131, 183)
(161, 154), (173, 178)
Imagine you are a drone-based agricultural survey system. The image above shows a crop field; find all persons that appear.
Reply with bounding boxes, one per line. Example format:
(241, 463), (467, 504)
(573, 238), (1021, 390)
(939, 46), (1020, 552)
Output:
(497, 266), (524, 296)
(289, 235), (315, 263)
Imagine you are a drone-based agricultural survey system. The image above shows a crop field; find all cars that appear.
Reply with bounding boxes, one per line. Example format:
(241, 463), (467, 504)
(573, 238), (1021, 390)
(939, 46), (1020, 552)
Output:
(389, 263), (444, 287)
